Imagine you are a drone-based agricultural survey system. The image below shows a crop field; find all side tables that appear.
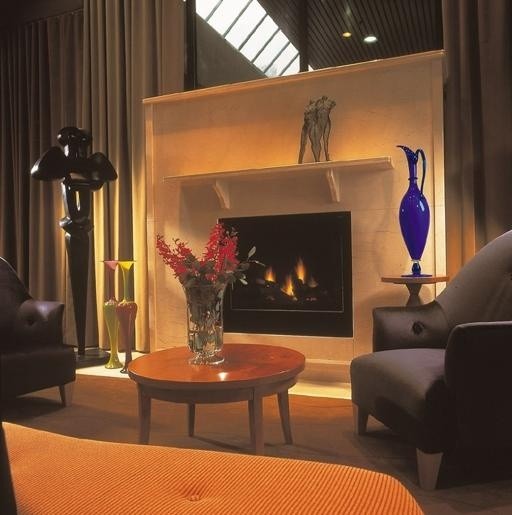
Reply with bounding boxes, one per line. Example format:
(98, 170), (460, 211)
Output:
(382, 276), (448, 307)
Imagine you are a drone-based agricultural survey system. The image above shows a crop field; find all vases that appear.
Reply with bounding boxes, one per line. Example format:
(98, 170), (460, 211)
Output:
(102, 261), (121, 368)
(183, 281), (226, 366)
(397, 145), (431, 276)
(116, 260), (137, 373)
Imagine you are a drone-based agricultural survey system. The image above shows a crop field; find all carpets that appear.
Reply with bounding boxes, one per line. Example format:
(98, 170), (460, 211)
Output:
(0, 374), (511, 514)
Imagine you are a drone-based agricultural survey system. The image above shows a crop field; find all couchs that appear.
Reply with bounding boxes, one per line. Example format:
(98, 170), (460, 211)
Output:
(0, 420), (427, 515)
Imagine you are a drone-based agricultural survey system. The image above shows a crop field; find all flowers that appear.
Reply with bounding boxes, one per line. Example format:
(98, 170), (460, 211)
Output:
(155, 221), (265, 348)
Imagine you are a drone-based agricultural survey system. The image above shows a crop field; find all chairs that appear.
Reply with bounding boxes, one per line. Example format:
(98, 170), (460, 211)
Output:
(1, 257), (75, 407)
(350, 230), (512, 493)
